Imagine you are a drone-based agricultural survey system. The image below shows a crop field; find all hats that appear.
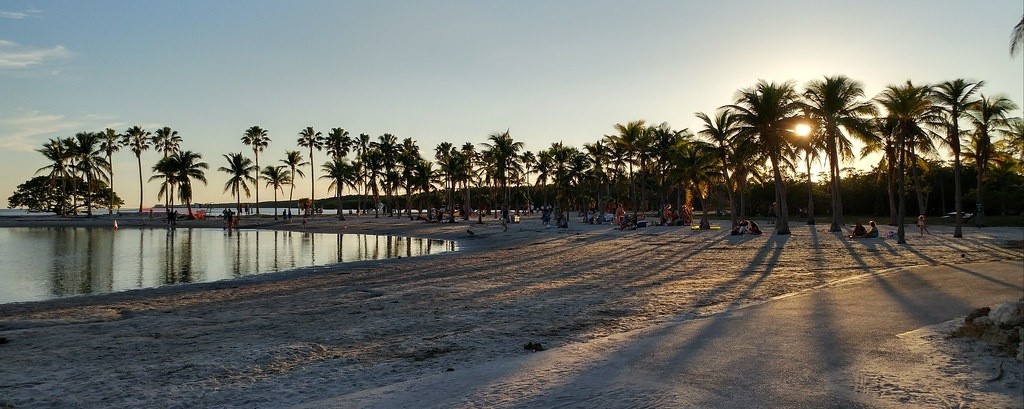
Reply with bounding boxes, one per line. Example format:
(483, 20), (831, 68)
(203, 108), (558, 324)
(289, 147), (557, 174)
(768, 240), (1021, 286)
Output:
(919, 215), (924, 220)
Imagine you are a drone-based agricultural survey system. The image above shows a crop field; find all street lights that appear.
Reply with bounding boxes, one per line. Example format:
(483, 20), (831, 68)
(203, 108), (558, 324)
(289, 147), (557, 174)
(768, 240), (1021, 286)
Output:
(976, 201), (982, 230)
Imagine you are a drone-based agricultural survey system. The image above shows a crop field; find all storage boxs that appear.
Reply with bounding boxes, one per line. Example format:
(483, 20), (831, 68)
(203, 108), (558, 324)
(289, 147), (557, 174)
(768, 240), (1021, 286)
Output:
(638, 221), (648, 227)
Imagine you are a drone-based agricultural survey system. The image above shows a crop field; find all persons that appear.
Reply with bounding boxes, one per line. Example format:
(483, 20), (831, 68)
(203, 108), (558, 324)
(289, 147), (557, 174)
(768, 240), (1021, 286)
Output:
(150, 208), (153, 219)
(499, 205), (511, 232)
(382, 205), (386, 215)
(117, 209), (120, 215)
(408, 207), (479, 224)
(222, 203), (250, 230)
(283, 205), (324, 229)
(917, 215), (930, 237)
(541, 208), (552, 225)
(587, 203), (625, 226)
(853, 221), (878, 237)
(167, 208), (178, 225)
(733, 219), (762, 234)
